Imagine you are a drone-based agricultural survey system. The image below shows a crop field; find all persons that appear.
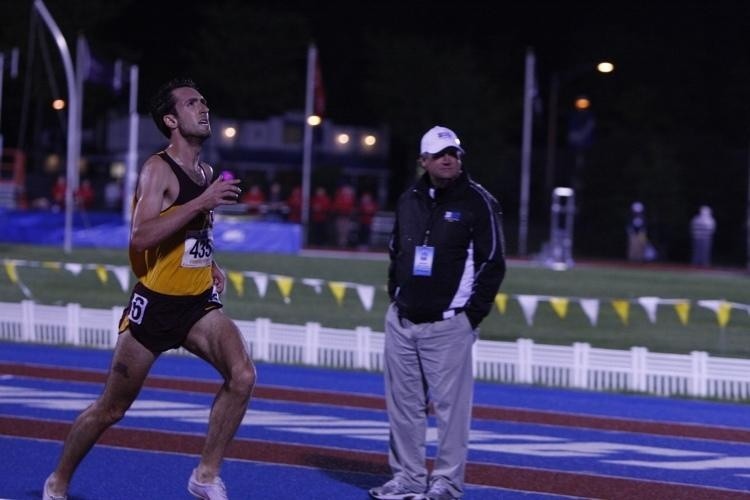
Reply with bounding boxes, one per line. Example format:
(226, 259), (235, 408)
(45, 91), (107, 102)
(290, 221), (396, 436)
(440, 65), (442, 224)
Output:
(368, 125), (506, 500)
(627, 202), (648, 259)
(40, 76), (256, 499)
(690, 206), (717, 267)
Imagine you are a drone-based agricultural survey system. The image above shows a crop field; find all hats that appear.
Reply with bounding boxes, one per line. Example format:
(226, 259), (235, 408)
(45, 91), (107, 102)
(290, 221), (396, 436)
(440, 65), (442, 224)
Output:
(419, 125), (465, 156)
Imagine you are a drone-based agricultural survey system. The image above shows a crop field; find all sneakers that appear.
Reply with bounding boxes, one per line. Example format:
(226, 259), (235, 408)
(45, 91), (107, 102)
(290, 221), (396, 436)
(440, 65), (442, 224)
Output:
(419, 478), (461, 500)
(369, 480), (423, 500)
(188, 467), (228, 500)
(42, 471), (67, 499)
